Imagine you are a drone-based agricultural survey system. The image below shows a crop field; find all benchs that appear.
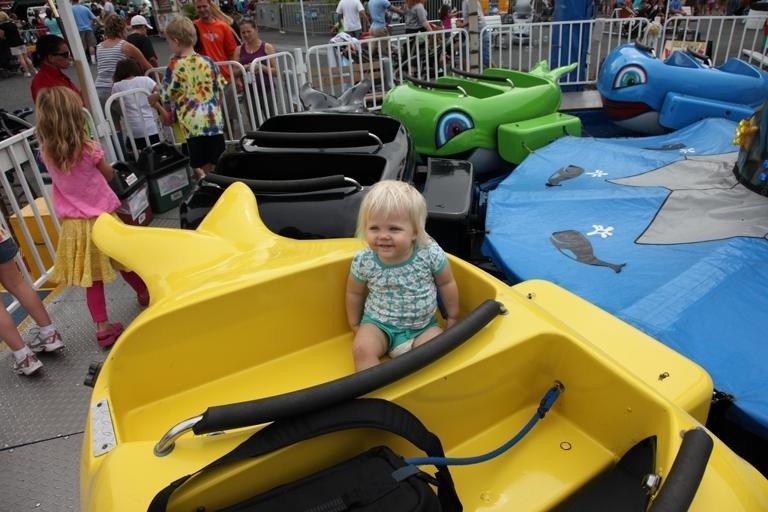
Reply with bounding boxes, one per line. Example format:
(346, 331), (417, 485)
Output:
(285, 58), (399, 110)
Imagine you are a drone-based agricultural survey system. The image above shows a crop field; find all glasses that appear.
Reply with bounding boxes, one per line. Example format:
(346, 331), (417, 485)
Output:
(238, 17), (252, 24)
(54, 51), (68, 59)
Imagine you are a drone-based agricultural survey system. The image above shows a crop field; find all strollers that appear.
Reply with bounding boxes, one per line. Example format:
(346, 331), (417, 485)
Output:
(329, 32), (449, 92)
(0, 106), (39, 183)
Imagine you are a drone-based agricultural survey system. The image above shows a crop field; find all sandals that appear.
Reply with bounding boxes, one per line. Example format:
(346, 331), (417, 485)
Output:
(96, 322), (123, 346)
(137, 291), (150, 306)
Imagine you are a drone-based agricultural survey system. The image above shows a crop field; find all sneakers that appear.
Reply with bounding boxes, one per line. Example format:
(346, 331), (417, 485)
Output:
(27, 325), (64, 353)
(23, 73), (30, 78)
(14, 354), (43, 375)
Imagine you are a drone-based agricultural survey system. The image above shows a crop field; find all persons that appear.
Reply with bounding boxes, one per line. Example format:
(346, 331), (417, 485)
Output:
(1, 0), (279, 186)
(327, 0), (767, 77)
(0, 210), (64, 377)
(33, 85), (149, 347)
(343, 180), (459, 374)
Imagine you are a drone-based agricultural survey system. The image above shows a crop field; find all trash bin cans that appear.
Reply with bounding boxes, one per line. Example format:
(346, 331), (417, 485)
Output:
(138, 140), (192, 216)
(107, 160), (154, 226)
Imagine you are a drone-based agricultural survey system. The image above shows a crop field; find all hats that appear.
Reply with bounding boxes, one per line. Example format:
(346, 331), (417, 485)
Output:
(131, 15), (153, 29)
